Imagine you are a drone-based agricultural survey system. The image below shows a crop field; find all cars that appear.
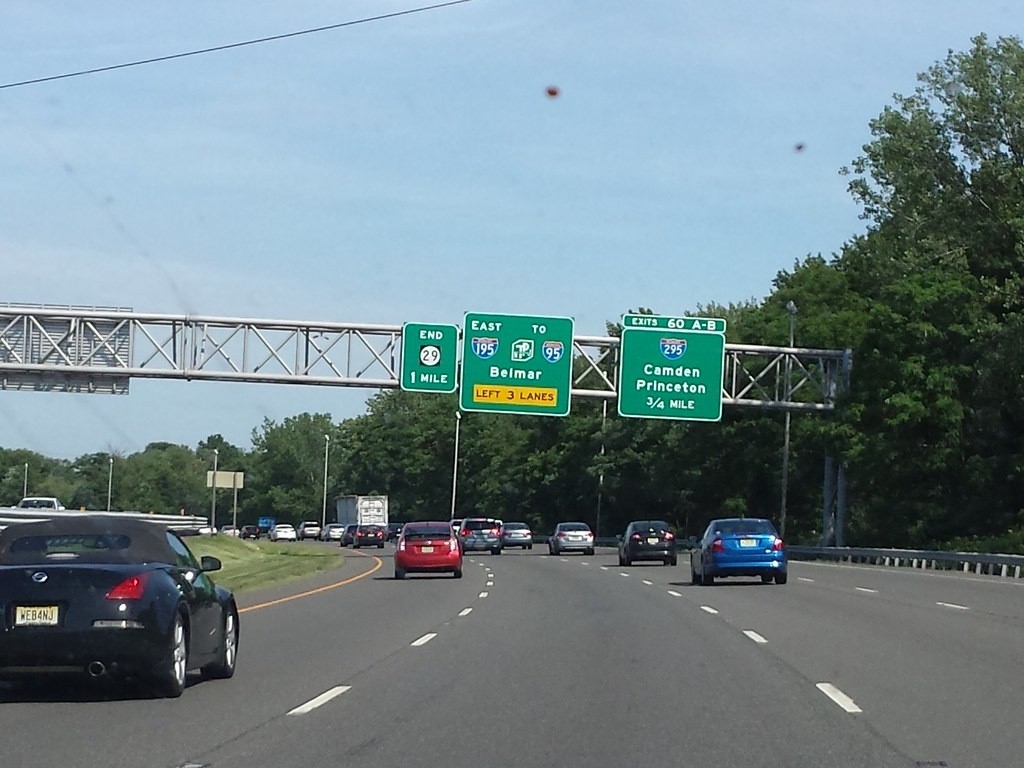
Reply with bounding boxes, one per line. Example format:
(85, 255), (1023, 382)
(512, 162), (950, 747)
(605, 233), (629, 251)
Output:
(324, 523), (345, 541)
(499, 522), (532, 550)
(395, 522), (464, 580)
(11, 496), (66, 511)
(0, 517), (240, 697)
(268, 524), (297, 542)
(616, 520), (676, 567)
(548, 522), (594, 556)
(687, 517), (788, 584)
(385, 522), (405, 541)
(449, 519), (464, 536)
(340, 526), (385, 549)
(241, 525), (261, 539)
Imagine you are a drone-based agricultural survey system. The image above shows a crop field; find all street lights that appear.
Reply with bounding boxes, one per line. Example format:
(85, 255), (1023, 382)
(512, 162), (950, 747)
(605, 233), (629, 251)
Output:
(23, 463), (29, 497)
(211, 447), (218, 530)
(451, 410), (462, 522)
(107, 459), (113, 511)
(323, 434), (329, 536)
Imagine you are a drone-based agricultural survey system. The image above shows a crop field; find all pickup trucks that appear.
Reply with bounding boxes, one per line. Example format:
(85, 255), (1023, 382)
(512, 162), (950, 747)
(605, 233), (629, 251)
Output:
(220, 525), (241, 536)
(298, 520), (321, 540)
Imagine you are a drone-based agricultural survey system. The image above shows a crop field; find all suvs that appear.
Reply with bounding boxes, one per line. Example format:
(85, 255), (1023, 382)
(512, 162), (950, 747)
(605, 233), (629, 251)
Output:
(457, 519), (504, 555)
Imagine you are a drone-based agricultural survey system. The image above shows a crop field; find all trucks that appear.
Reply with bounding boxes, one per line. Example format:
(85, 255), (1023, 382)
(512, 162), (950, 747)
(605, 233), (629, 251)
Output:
(334, 494), (388, 528)
